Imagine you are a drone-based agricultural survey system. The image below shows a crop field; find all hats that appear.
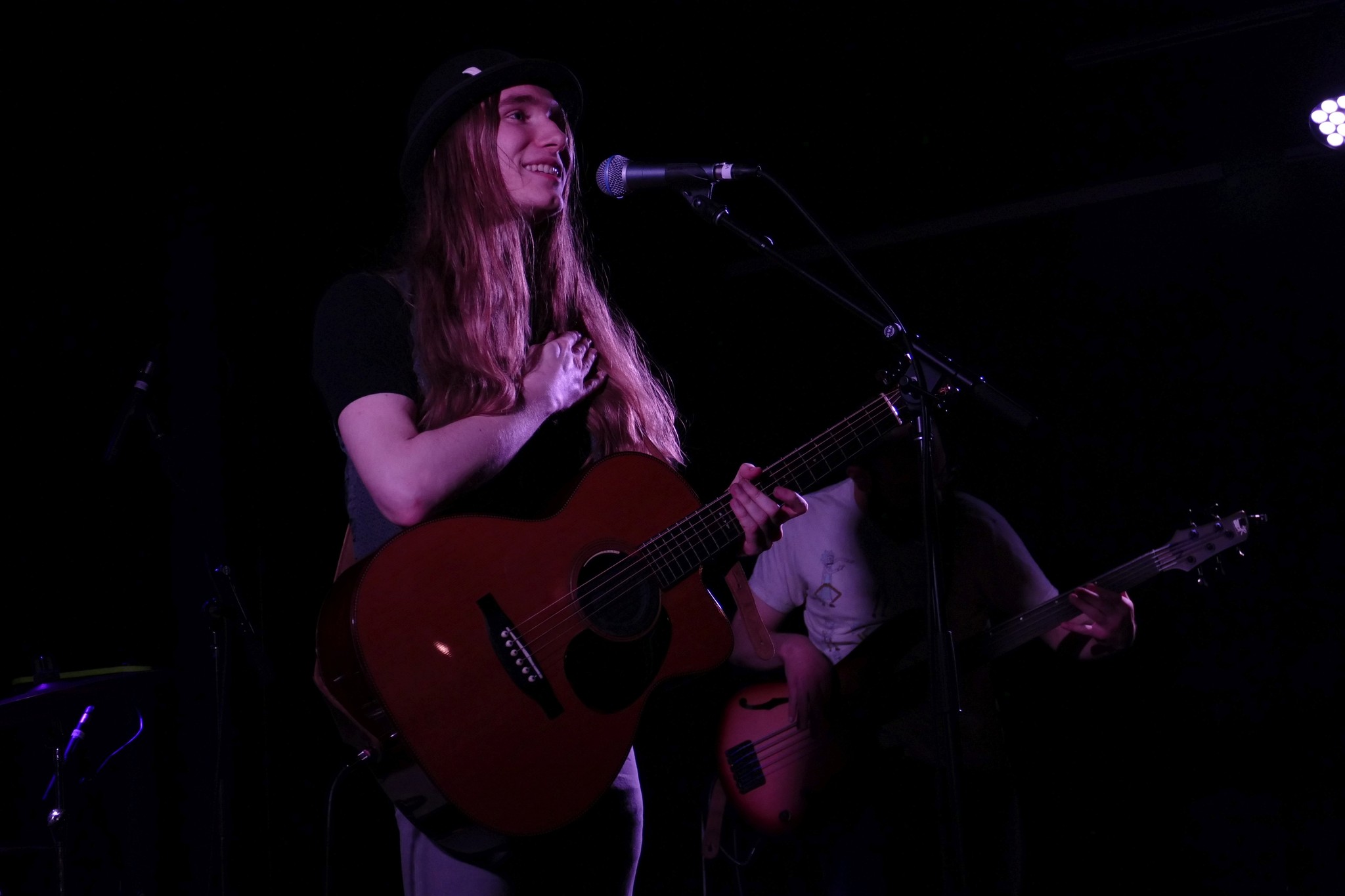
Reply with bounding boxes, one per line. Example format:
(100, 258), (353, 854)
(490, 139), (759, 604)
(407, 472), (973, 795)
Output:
(401, 50), (584, 202)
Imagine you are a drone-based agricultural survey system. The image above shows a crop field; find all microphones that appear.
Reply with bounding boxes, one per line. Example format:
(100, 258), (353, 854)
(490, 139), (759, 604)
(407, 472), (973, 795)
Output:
(64, 705), (97, 762)
(596, 155), (762, 199)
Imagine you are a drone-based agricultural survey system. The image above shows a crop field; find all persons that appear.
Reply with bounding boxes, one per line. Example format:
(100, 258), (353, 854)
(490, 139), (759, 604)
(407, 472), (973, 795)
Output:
(729, 466), (1139, 896)
(311, 49), (811, 896)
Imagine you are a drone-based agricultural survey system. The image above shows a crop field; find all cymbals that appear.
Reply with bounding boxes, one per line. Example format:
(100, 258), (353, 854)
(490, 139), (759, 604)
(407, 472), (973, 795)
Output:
(0, 672), (139, 708)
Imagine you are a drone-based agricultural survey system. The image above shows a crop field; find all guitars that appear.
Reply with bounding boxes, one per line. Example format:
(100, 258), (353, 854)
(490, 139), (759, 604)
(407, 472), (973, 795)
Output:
(318, 350), (997, 873)
(966, 499), (1268, 663)
